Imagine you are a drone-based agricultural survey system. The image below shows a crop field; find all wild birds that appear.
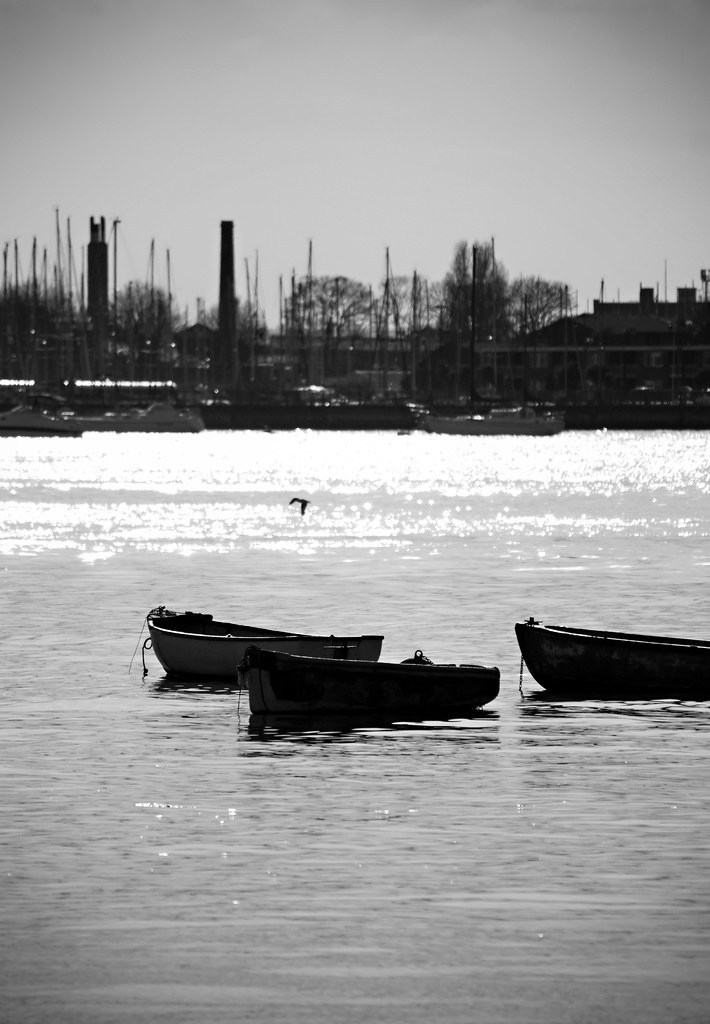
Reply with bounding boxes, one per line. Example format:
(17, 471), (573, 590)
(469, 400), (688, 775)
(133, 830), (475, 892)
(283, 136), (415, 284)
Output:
(289, 497), (310, 516)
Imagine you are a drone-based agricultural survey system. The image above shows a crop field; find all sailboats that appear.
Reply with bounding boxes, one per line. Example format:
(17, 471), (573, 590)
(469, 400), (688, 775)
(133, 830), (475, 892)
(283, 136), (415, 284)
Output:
(1, 207), (594, 432)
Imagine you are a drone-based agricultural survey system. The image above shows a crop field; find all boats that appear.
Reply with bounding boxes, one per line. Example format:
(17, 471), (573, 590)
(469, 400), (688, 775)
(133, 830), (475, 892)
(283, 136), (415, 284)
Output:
(74, 400), (206, 433)
(236, 643), (502, 723)
(138, 601), (388, 692)
(426, 396), (568, 437)
(0, 388), (81, 438)
(512, 617), (710, 704)
